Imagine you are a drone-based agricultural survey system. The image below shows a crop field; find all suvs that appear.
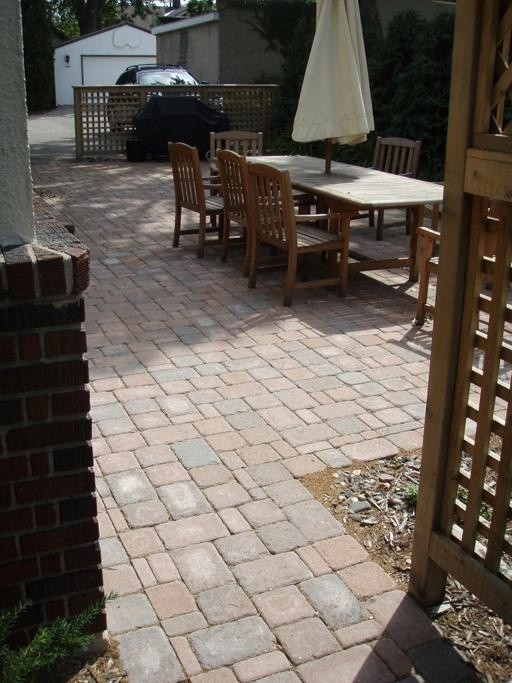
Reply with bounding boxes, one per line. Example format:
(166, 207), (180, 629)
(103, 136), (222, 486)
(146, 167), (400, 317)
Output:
(107, 61), (226, 131)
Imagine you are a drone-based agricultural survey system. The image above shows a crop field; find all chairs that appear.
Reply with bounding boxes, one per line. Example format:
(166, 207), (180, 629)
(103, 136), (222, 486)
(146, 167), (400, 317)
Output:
(350, 136), (422, 239)
(167, 141), (243, 259)
(409, 180), (444, 249)
(210, 131), (264, 227)
(240, 154), (361, 306)
(216, 150), (286, 277)
(415, 209), (501, 328)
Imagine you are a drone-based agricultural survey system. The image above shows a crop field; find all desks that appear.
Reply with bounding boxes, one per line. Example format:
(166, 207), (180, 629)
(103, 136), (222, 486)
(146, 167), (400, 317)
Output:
(211, 154), (445, 297)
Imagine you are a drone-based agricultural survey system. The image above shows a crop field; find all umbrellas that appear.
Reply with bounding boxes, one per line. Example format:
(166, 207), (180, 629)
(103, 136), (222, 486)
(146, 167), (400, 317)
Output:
(291, 0), (375, 174)
